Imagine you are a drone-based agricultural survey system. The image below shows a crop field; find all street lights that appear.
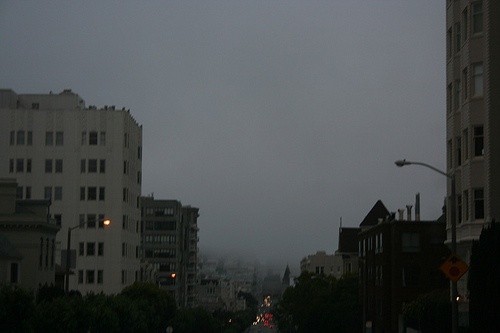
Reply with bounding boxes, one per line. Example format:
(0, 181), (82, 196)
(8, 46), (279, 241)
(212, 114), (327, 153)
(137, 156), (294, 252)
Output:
(394, 158), (462, 333)
(65, 218), (112, 294)
